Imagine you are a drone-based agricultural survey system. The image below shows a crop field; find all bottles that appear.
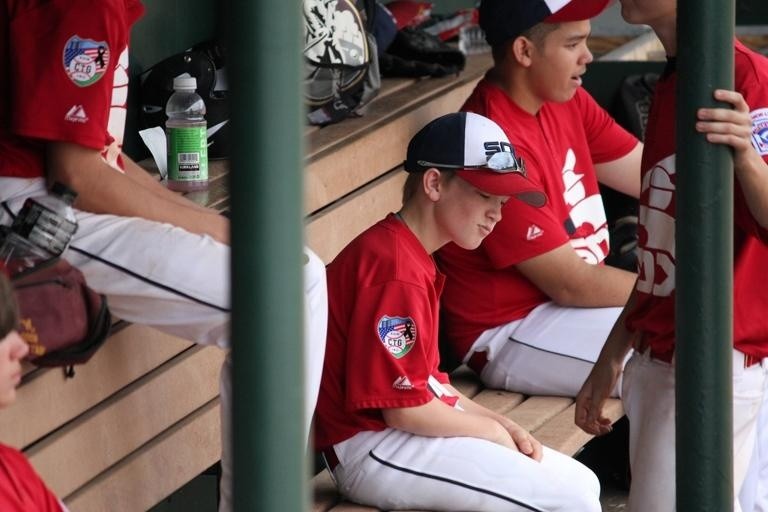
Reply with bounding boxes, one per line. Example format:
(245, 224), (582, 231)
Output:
(164, 75), (208, 193)
(0, 180), (84, 274)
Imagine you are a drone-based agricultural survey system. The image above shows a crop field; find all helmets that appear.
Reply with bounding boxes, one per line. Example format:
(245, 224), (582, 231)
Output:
(135, 38), (232, 157)
(356, 0), (469, 78)
(302, 0), (370, 126)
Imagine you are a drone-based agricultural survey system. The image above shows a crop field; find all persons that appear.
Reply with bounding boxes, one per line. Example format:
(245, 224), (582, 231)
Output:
(574, 1), (767, 512)
(303, 111), (602, 512)
(1, 2), (330, 512)
(435, 1), (645, 399)
(0, 267), (75, 512)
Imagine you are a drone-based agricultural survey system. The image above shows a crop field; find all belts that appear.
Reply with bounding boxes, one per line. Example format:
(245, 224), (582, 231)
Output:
(323, 445), (339, 472)
(466, 350), (486, 376)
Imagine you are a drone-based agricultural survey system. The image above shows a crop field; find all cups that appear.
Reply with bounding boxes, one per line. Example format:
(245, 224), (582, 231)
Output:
(458, 25), (484, 56)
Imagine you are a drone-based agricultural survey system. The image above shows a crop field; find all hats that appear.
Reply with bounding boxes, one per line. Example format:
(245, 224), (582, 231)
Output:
(402, 111), (547, 208)
(478, 0), (610, 24)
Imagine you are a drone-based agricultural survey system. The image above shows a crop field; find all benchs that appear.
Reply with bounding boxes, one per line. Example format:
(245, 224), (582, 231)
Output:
(0, 51), (496, 512)
(307, 376), (628, 512)
